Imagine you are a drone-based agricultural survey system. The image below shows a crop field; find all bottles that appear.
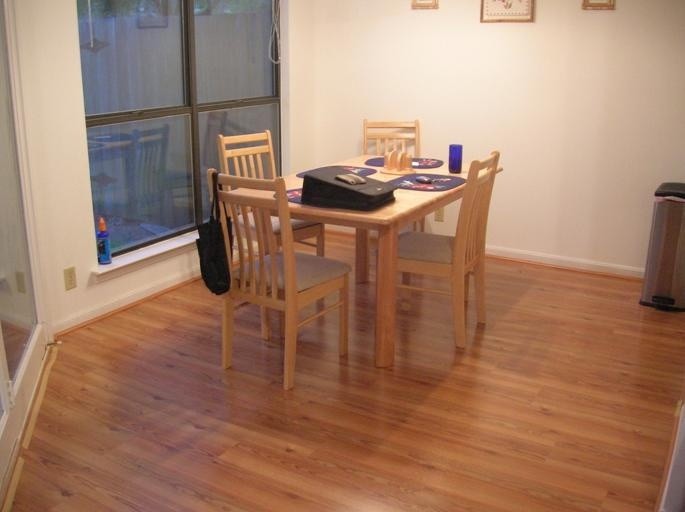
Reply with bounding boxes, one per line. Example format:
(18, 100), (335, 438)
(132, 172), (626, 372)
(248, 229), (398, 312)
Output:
(97, 218), (112, 265)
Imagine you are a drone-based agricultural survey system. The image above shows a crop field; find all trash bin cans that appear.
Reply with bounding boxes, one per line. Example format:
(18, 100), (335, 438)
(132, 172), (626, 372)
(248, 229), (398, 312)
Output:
(639, 182), (685, 311)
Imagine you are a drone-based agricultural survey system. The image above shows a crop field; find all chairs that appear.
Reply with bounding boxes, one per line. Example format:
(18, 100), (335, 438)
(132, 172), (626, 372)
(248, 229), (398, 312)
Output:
(372, 149), (500, 351)
(357, 118), (424, 289)
(205, 167), (353, 391)
(215, 128), (329, 310)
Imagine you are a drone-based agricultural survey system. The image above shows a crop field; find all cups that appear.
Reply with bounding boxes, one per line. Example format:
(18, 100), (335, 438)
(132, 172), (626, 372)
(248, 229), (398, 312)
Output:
(449, 144), (463, 173)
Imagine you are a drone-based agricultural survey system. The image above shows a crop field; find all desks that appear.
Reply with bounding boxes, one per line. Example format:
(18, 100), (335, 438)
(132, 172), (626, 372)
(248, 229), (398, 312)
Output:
(239, 151), (488, 371)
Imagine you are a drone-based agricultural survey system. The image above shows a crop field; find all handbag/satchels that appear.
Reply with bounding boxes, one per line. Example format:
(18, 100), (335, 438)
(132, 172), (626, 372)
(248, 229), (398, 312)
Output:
(301, 166), (398, 211)
(196, 172), (234, 295)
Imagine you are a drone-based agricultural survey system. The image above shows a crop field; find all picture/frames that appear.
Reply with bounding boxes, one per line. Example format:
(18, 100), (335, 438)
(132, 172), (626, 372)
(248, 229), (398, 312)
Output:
(481, 0), (536, 23)
